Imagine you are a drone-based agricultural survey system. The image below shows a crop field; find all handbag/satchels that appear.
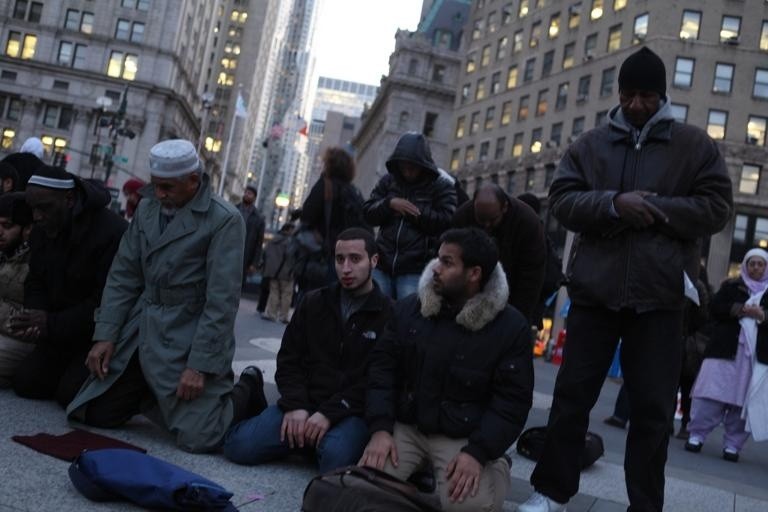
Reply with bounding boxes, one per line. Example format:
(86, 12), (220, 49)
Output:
(301, 465), (440, 512)
(286, 176), (333, 284)
(68, 447), (239, 511)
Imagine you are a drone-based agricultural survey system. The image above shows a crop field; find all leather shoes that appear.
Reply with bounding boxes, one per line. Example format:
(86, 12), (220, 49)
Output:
(604, 416), (626, 429)
(723, 446), (739, 462)
(685, 437), (702, 451)
(240, 366), (269, 410)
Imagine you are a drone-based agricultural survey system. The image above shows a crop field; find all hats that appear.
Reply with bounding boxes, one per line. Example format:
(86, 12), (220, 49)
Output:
(617, 46), (666, 97)
(28, 165), (76, 190)
(150, 139), (199, 179)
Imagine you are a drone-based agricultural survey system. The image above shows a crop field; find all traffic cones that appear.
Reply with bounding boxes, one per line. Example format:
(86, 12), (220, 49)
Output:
(550, 329), (567, 365)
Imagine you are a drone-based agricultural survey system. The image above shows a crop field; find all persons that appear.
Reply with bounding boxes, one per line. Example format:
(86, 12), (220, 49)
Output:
(670, 265), (714, 436)
(685, 247), (766, 464)
(222, 228), (397, 473)
(602, 278), (713, 439)
(531, 46), (734, 511)
(362, 132), (458, 302)
(236, 186), (265, 291)
(452, 180), (545, 328)
(293, 147), (364, 304)
(123, 178), (146, 223)
(66, 139), (269, 452)
(358, 226), (534, 509)
(256, 208), (302, 324)
(3, 152), (131, 410)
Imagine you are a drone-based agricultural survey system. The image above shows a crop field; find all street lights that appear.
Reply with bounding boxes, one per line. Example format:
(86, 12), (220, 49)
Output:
(273, 192), (290, 232)
(91, 80), (142, 185)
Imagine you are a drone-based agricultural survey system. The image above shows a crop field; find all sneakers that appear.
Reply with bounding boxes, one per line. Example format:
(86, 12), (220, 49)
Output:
(518, 491), (567, 511)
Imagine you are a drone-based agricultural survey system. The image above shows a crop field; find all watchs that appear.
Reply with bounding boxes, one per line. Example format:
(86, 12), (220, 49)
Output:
(193, 368), (203, 375)
